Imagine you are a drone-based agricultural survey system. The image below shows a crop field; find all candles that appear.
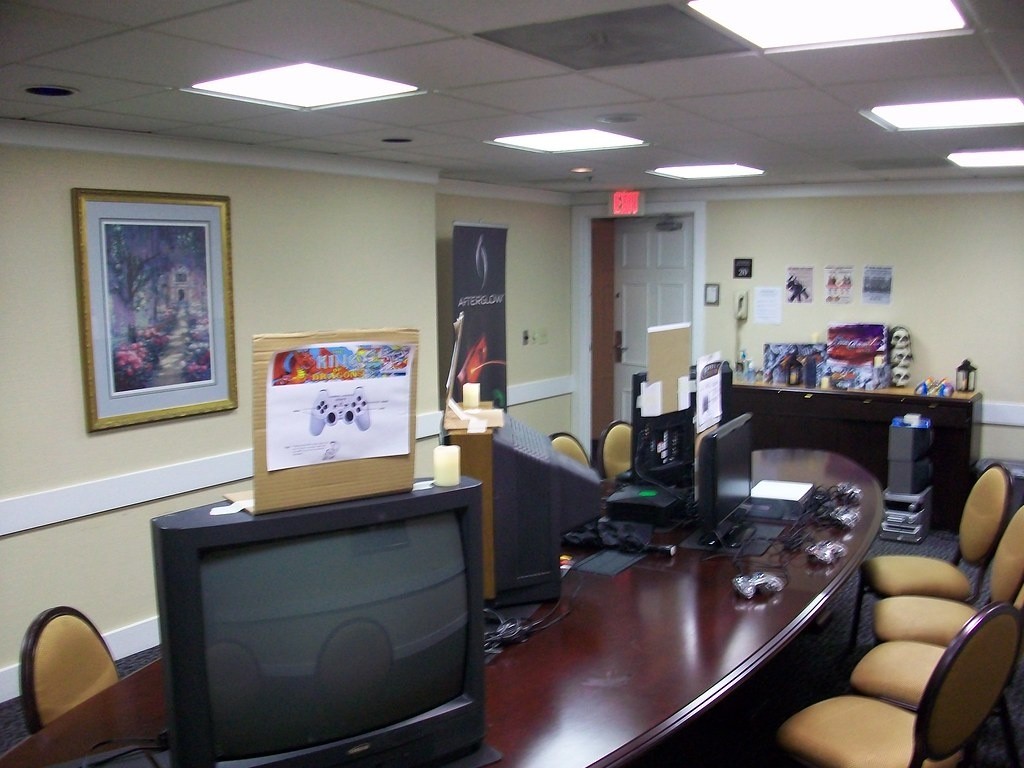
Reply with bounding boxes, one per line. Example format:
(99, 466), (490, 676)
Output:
(434, 445), (461, 486)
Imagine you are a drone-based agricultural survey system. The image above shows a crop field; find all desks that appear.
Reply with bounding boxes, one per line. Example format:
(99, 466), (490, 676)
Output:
(733, 374), (982, 535)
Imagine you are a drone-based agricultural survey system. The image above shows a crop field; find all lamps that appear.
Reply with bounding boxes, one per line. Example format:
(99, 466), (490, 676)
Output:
(955, 359), (978, 392)
(655, 213), (683, 232)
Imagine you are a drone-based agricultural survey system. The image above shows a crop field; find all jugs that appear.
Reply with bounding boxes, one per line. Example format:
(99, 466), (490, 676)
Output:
(955, 359), (976, 392)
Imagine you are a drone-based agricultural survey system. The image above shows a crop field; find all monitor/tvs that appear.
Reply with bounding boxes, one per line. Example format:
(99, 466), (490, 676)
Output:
(437, 406), (608, 608)
(148, 476), (507, 768)
(698, 412), (758, 552)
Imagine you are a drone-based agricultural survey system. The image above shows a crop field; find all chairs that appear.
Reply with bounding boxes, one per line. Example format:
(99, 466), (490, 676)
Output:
(777, 601), (1024, 768)
(847, 461), (1013, 648)
(850, 584), (1024, 712)
(874, 504), (1024, 647)
(547, 431), (589, 467)
(0, 445), (884, 768)
(597, 419), (632, 477)
(18, 605), (122, 734)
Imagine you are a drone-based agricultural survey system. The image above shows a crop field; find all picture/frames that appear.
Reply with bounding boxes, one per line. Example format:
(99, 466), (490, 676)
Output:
(70, 187), (238, 433)
